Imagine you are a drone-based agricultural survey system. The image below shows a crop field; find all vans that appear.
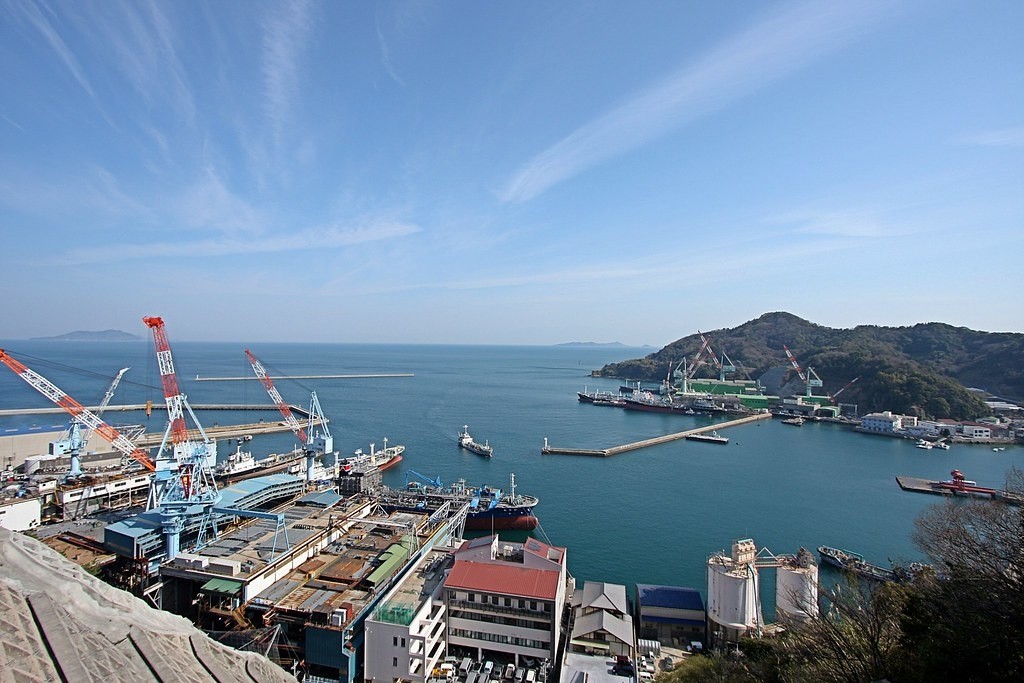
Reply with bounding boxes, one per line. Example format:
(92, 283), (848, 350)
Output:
(428, 655), (543, 683)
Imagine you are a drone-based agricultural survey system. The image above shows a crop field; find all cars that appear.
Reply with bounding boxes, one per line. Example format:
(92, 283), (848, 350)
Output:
(638, 650), (656, 683)
(611, 655), (635, 678)
(665, 657), (672, 671)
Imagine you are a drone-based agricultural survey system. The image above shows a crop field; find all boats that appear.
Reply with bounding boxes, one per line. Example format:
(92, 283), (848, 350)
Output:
(575, 355), (727, 416)
(457, 424), (493, 458)
(781, 416), (805, 426)
(915, 438), (951, 450)
(353, 471), (539, 533)
(816, 544), (897, 584)
